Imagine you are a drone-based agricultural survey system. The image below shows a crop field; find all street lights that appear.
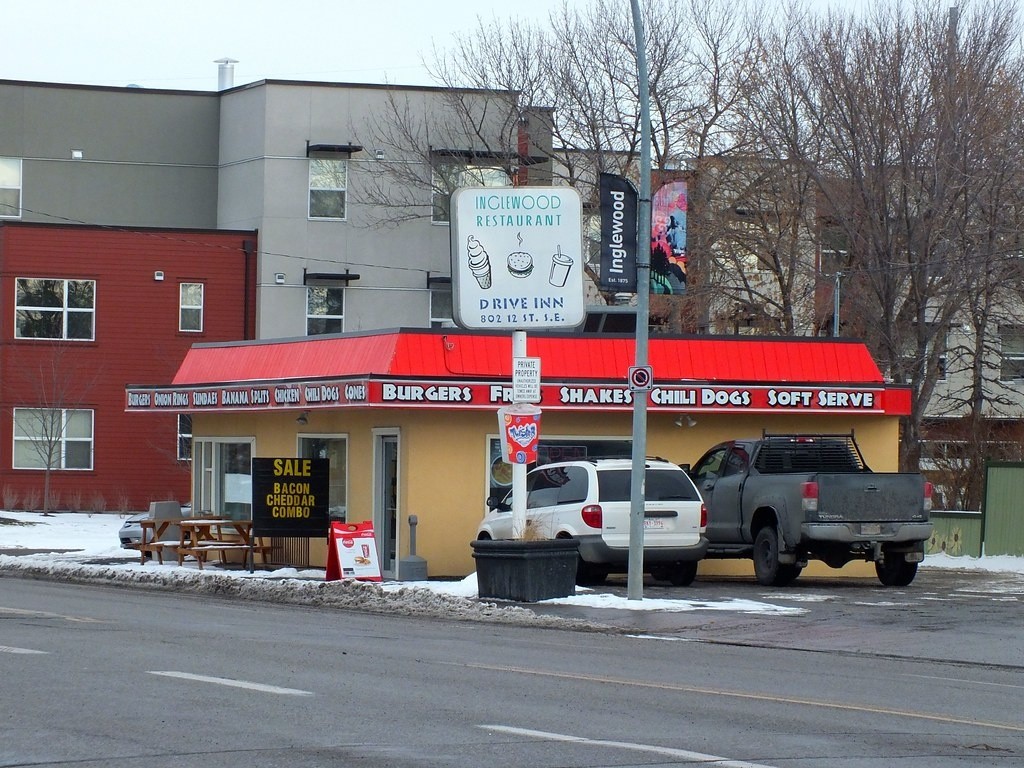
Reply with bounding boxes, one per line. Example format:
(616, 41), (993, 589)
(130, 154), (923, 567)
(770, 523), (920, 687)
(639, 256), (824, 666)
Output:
(820, 249), (848, 338)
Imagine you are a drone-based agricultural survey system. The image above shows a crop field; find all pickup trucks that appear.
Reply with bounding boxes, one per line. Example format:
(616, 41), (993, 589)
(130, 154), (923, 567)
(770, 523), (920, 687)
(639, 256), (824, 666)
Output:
(676, 427), (934, 588)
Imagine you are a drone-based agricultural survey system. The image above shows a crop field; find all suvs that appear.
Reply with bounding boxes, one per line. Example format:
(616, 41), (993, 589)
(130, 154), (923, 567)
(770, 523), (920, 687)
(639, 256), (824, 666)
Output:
(474, 453), (710, 587)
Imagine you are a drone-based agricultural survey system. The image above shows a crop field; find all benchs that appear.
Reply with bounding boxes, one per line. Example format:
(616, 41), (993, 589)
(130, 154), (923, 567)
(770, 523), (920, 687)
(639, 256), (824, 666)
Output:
(126, 539), (286, 558)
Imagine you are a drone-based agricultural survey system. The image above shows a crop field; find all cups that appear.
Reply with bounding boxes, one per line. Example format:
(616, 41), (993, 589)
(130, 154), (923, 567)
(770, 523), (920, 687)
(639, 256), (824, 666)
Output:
(503, 410), (542, 464)
(362, 545), (369, 557)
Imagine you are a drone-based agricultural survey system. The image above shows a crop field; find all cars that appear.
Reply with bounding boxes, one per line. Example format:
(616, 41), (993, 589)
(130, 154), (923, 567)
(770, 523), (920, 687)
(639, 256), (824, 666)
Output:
(118, 500), (191, 558)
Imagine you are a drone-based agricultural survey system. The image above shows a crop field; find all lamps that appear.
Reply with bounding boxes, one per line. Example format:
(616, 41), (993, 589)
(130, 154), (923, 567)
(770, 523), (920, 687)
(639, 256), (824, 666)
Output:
(155, 271), (164, 280)
(276, 273), (285, 284)
(375, 150), (384, 160)
(676, 413), (697, 427)
(296, 410), (309, 425)
(72, 150), (83, 160)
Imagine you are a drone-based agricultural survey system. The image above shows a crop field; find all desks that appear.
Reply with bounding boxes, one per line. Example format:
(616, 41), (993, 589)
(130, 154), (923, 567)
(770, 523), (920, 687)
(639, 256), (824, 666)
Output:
(177, 520), (267, 569)
(140, 515), (234, 565)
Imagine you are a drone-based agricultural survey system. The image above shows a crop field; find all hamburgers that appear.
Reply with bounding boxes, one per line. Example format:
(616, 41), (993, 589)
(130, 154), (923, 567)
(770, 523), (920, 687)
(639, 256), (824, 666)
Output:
(354, 557), (364, 564)
